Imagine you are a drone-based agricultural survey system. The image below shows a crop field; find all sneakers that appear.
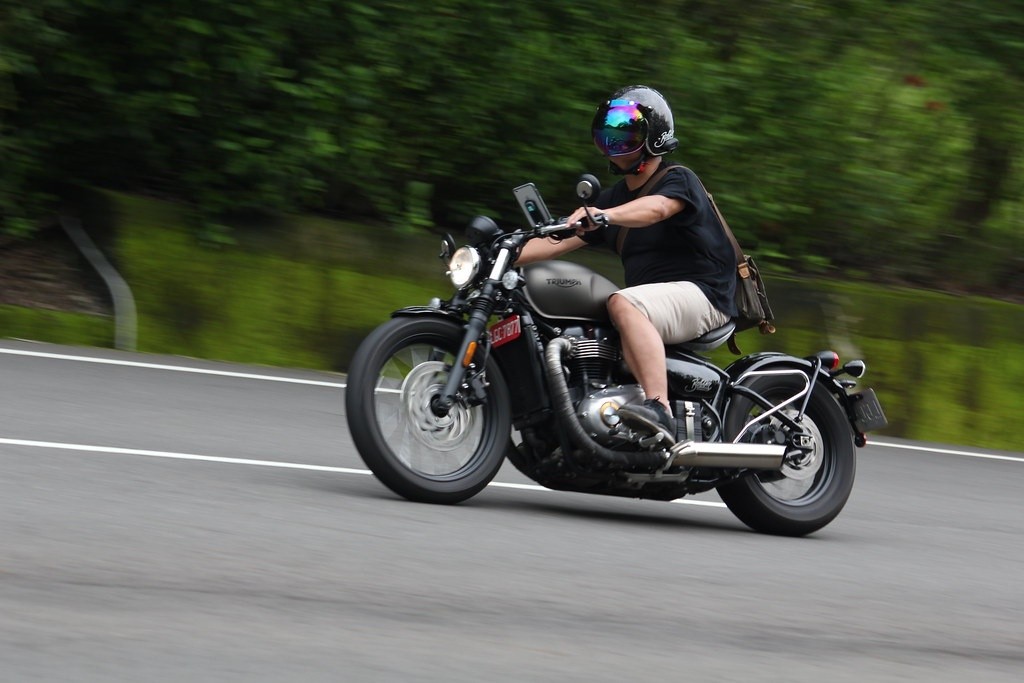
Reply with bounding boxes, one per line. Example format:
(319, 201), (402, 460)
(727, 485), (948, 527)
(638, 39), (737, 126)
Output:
(618, 396), (677, 450)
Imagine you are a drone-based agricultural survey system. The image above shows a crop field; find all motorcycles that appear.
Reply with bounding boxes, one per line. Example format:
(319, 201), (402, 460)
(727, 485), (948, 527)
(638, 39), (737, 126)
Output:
(345, 175), (889, 537)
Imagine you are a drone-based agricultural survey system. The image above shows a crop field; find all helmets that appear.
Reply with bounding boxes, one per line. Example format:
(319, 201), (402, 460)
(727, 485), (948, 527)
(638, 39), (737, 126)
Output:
(592, 85), (679, 158)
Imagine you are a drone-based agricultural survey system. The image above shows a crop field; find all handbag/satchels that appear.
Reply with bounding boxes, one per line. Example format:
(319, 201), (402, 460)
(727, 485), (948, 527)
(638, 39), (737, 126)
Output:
(728, 255), (774, 333)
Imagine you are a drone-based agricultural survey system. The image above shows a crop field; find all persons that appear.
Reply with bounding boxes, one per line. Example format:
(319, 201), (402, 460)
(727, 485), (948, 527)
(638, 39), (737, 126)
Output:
(511, 85), (740, 445)
